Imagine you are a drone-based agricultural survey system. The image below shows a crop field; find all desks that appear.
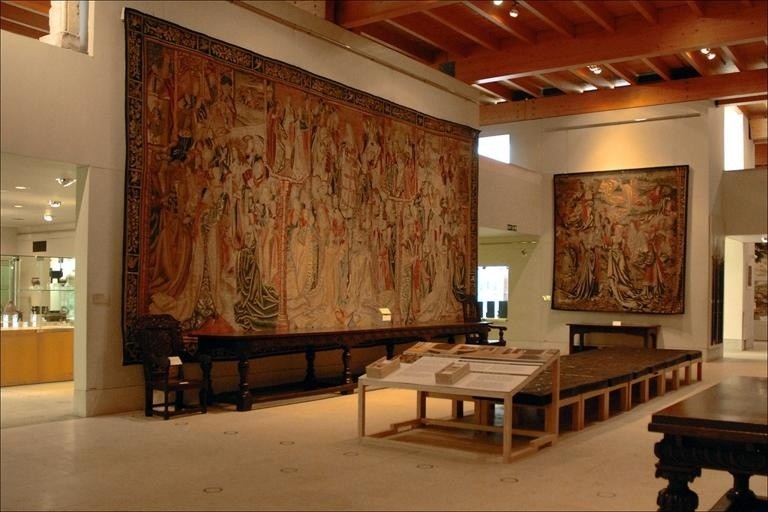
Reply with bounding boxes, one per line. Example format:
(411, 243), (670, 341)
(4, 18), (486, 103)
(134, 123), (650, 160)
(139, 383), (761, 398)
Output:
(648, 374), (767, 512)
(189, 321), (495, 411)
(566, 321), (661, 353)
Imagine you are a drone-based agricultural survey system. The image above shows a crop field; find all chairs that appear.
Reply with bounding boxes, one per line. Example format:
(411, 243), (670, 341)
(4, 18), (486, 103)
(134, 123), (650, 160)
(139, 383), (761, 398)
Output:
(140, 313), (210, 420)
(461, 299), (507, 345)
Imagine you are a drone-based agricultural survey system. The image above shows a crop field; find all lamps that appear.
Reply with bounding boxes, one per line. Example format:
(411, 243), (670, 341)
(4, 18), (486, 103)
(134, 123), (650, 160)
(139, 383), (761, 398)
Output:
(509, 2), (519, 19)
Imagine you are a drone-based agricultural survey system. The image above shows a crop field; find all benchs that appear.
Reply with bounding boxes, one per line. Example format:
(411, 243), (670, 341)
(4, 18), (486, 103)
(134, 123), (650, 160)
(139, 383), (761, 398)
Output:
(424, 347), (703, 452)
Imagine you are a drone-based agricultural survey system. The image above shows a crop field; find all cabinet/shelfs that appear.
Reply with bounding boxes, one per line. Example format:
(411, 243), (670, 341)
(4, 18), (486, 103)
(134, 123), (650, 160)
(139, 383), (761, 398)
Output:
(20, 254), (76, 324)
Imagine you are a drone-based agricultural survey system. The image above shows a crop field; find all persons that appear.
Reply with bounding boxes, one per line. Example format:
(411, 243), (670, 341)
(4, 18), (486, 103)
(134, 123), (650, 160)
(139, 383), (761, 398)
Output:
(556, 174), (675, 308)
